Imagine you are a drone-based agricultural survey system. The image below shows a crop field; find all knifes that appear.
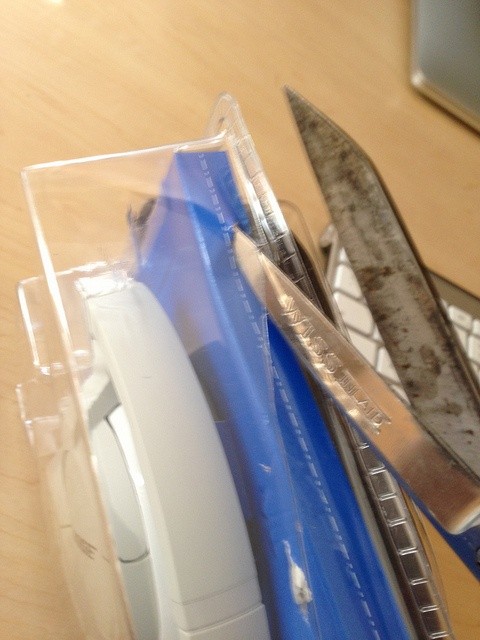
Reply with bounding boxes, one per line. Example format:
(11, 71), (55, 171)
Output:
(233, 228), (479, 584)
(282, 84), (480, 479)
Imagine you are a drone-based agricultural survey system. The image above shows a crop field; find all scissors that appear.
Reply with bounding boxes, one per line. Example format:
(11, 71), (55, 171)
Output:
(235, 84), (480, 579)
